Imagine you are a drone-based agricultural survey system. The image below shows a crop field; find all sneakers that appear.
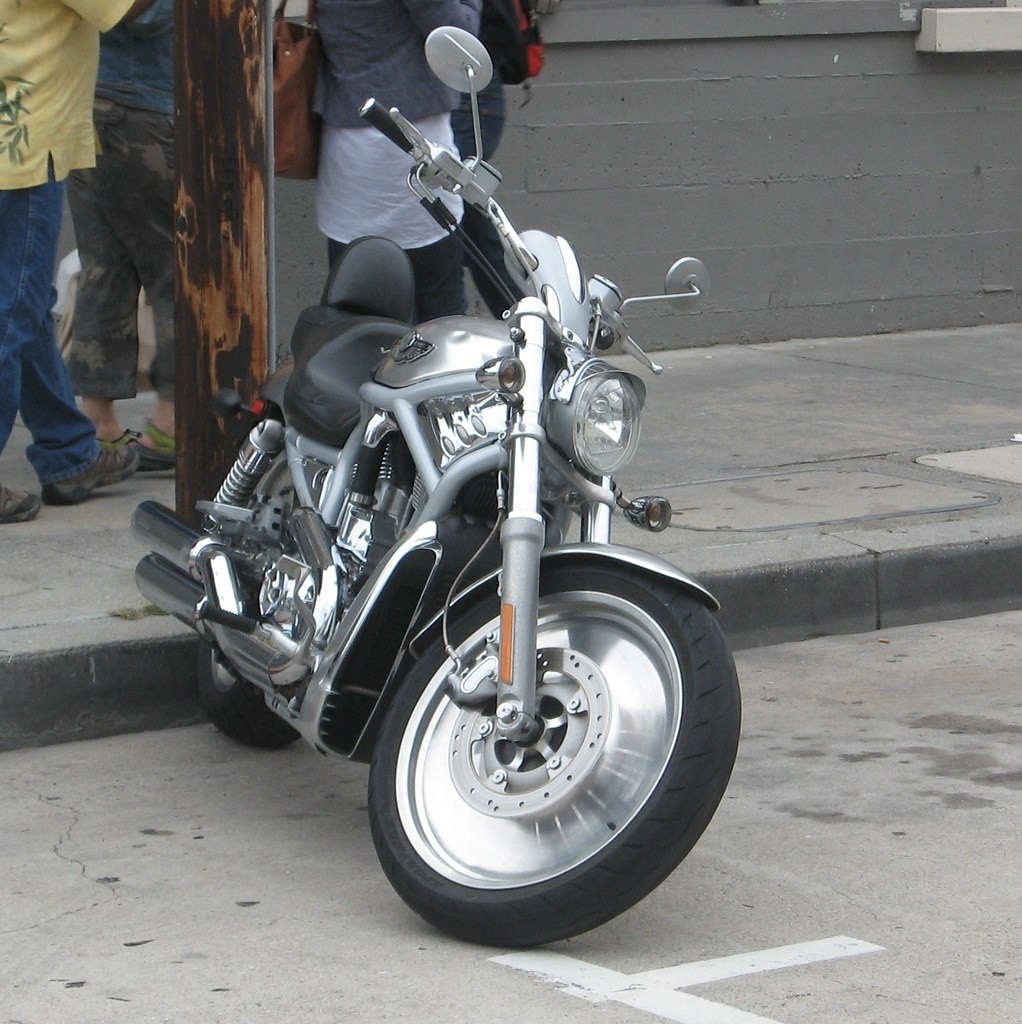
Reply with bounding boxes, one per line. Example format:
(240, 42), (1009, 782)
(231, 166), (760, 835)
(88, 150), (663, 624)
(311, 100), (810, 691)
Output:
(43, 444), (140, 504)
(0, 486), (39, 524)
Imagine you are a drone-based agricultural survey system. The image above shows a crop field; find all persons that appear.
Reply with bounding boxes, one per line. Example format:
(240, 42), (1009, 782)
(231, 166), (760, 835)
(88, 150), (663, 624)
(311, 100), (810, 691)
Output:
(62, 0), (182, 472)
(447, 33), (533, 316)
(306, 0), (489, 319)
(1, 0), (162, 523)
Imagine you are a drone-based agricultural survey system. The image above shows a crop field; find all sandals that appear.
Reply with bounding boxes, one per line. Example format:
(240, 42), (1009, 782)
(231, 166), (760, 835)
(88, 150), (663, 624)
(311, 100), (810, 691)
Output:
(95, 417), (176, 472)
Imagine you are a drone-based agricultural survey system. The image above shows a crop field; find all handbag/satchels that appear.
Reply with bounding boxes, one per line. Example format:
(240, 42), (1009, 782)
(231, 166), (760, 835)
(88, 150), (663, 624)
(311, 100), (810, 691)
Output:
(274, 1), (319, 179)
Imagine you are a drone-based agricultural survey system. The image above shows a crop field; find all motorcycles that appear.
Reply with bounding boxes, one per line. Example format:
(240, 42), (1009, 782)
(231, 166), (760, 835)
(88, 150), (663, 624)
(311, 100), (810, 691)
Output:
(129, 23), (745, 948)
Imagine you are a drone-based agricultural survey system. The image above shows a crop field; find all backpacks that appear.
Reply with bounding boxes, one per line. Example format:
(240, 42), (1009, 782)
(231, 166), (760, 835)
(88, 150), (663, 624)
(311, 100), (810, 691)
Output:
(478, 0), (544, 86)
